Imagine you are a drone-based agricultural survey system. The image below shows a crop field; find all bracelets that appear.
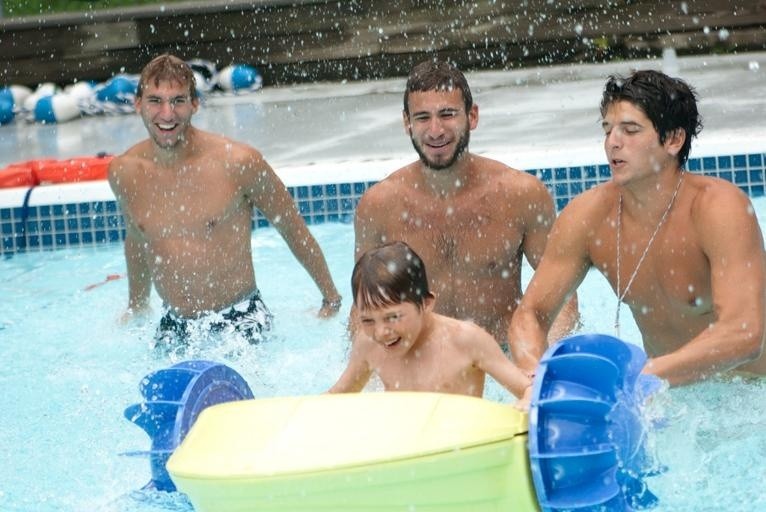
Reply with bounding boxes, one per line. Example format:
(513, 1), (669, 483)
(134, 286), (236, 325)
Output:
(320, 294), (341, 313)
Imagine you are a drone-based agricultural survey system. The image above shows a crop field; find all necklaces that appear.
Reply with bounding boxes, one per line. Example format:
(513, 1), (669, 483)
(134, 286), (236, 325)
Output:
(614, 168), (688, 336)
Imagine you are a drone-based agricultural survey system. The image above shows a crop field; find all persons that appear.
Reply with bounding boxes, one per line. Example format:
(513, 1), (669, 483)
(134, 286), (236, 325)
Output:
(316, 242), (534, 410)
(105, 52), (343, 356)
(508, 66), (766, 391)
(352, 55), (581, 358)
